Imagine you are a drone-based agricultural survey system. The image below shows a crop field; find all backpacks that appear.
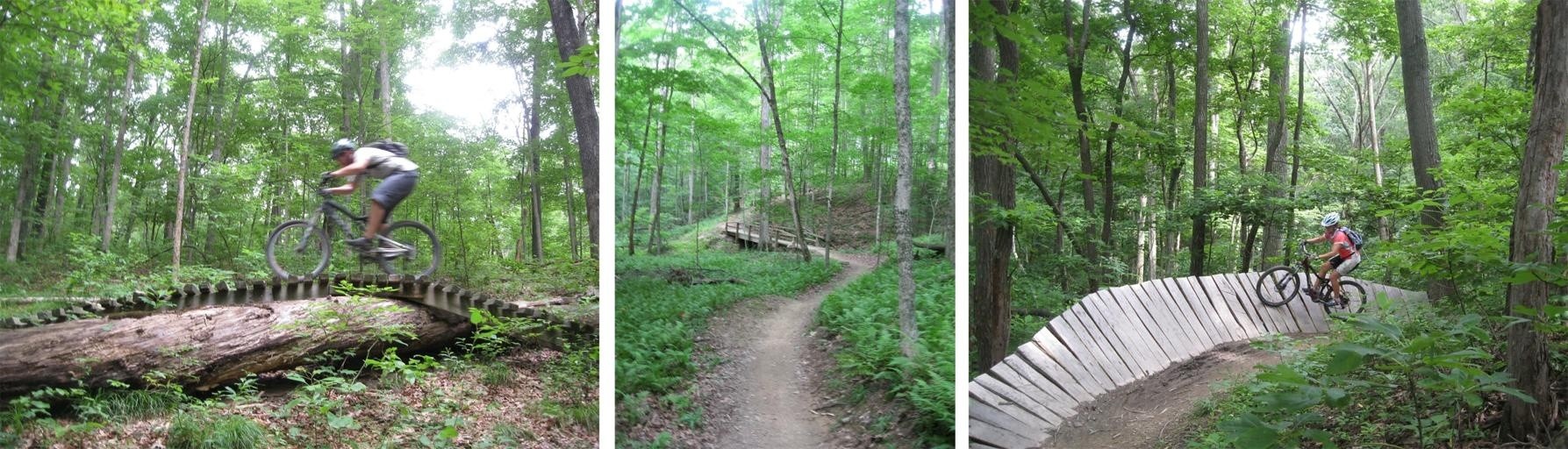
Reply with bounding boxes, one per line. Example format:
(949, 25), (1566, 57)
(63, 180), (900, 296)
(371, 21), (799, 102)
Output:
(364, 141), (408, 156)
(1339, 226), (1363, 250)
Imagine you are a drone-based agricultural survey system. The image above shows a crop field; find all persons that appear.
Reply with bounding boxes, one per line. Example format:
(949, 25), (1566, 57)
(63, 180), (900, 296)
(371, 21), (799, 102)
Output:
(1300, 212), (1362, 306)
(320, 136), (421, 259)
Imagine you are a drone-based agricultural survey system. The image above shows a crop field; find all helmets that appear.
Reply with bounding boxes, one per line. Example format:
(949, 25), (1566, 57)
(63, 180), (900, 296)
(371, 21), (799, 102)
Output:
(332, 138), (354, 158)
(1321, 212), (1340, 226)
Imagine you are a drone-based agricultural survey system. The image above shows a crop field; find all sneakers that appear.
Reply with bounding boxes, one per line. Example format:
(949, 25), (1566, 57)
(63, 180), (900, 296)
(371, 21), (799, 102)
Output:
(347, 237), (371, 250)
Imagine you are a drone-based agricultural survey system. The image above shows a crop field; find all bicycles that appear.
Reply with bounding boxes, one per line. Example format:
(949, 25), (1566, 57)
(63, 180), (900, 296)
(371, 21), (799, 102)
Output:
(1255, 239), (1367, 322)
(266, 171), (442, 282)
